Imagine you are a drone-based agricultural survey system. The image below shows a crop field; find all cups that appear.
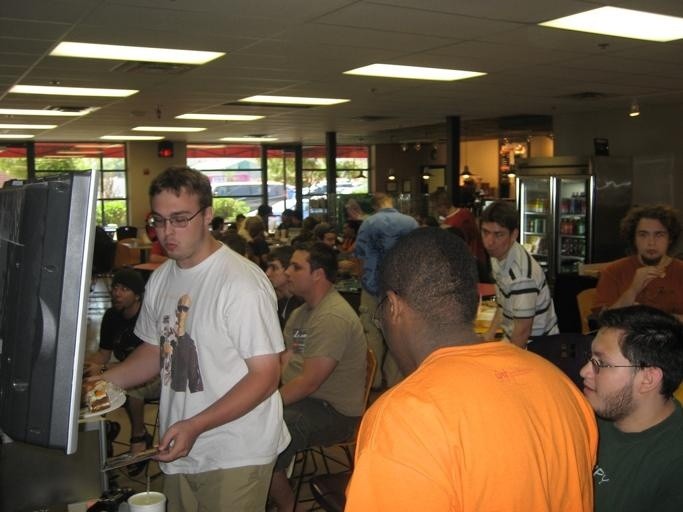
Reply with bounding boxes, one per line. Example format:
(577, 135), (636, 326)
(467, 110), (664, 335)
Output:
(127, 491), (168, 511)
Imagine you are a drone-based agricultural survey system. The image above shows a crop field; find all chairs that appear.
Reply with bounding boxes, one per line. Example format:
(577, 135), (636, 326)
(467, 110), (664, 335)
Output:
(577, 287), (594, 333)
(282, 350), (378, 512)
(103, 399), (162, 491)
(307, 468), (352, 512)
(117, 226), (138, 240)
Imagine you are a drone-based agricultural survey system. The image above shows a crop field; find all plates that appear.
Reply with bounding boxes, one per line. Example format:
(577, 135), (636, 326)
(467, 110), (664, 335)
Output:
(79, 384), (127, 419)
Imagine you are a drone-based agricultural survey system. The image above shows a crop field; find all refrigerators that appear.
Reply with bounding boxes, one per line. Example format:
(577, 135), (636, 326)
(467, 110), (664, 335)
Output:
(512, 155), (633, 293)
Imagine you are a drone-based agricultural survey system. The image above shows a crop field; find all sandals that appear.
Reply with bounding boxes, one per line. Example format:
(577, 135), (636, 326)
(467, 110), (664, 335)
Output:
(127, 433), (153, 476)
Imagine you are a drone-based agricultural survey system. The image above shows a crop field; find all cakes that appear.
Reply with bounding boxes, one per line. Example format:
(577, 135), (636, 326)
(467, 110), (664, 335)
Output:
(79, 378), (112, 414)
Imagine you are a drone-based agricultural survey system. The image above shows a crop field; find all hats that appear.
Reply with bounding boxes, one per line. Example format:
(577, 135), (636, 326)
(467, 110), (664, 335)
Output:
(113, 270), (145, 293)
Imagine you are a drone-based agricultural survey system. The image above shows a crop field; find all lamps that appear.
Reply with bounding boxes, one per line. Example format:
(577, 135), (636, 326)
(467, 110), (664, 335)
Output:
(459, 142), (473, 178)
(420, 149), (433, 179)
(629, 104), (640, 117)
(158, 141), (173, 157)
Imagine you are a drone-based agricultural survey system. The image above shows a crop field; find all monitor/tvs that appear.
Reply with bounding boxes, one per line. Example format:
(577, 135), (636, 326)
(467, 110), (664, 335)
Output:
(0, 169), (100, 455)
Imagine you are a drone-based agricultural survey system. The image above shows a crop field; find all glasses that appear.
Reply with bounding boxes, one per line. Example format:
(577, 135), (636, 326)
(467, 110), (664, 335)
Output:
(584, 352), (640, 374)
(372, 291), (397, 328)
(146, 207), (205, 228)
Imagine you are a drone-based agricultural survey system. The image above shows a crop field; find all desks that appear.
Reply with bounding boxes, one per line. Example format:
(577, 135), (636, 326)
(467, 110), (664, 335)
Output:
(125, 261), (160, 273)
(117, 238), (151, 266)
(474, 301), (504, 340)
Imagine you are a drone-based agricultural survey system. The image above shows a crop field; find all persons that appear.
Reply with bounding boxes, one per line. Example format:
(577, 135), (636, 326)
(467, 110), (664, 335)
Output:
(83, 167), (682, 511)
